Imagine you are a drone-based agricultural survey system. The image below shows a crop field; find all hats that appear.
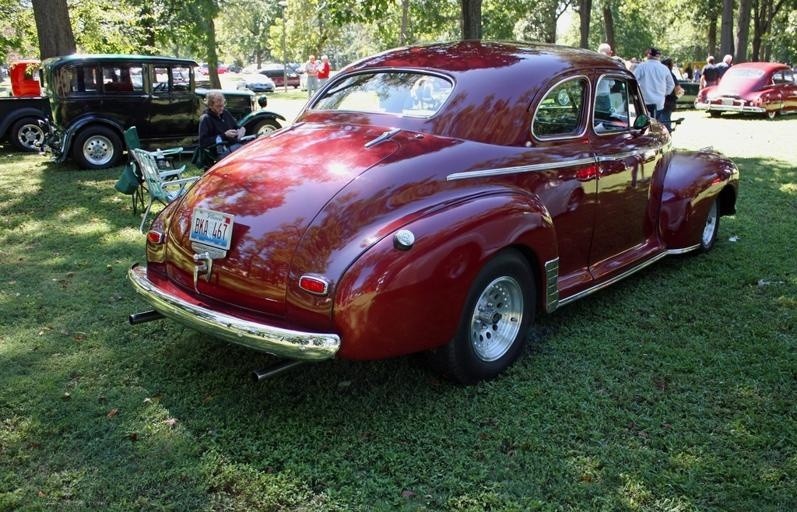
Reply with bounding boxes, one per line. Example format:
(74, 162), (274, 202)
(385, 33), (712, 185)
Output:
(648, 48), (662, 57)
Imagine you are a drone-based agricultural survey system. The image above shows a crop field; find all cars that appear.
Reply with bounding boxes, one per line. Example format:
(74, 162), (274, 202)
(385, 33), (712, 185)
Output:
(692, 61), (797, 120)
(0, 54), (300, 170)
(125, 36), (743, 390)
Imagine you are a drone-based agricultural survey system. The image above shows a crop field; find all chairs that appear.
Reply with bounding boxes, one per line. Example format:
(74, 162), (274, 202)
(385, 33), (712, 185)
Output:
(124, 126), (186, 213)
(199, 141), (232, 173)
(132, 148), (201, 232)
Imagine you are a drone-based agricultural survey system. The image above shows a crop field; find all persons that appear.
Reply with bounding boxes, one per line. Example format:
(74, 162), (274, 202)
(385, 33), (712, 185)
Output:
(598, 43), (733, 133)
(199, 92), (246, 161)
(306, 55), (319, 98)
(316, 56), (330, 86)
